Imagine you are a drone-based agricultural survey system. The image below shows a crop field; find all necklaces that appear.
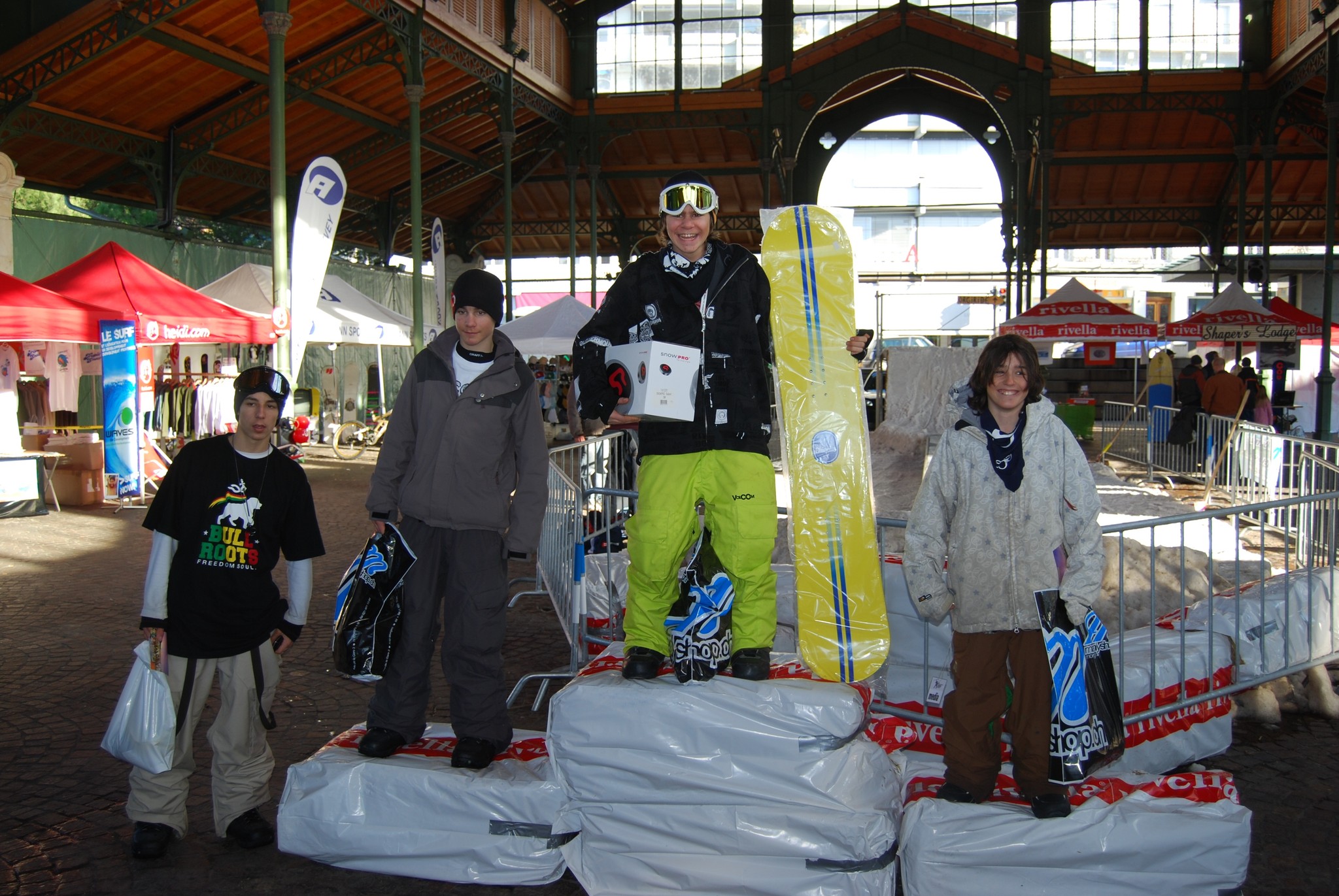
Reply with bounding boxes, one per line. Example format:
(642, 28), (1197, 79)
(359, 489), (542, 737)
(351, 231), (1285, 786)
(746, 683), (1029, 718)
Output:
(232, 433), (271, 534)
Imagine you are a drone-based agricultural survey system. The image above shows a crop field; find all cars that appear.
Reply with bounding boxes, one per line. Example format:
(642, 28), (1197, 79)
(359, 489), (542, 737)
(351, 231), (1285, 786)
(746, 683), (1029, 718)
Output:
(1060, 341), (1170, 358)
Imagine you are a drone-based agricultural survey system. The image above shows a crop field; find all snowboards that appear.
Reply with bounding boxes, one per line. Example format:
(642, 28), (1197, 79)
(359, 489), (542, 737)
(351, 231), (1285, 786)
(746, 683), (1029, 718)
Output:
(762, 205), (890, 685)
(1149, 351), (1173, 441)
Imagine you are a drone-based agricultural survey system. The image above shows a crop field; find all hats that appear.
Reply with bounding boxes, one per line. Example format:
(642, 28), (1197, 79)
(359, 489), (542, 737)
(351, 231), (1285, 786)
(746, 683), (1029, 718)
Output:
(526, 355), (575, 425)
(663, 171), (714, 191)
(234, 365), (286, 427)
(1167, 349), (1176, 355)
(1242, 357), (1251, 366)
(452, 268), (504, 328)
(1190, 355), (1202, 365)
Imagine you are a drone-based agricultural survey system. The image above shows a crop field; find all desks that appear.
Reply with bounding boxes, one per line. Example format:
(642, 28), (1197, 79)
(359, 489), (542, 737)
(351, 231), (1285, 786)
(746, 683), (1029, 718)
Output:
(0, 450), (66, 512)
(1271, 404), (1303, 435)
(1053, 404), (1095, 442)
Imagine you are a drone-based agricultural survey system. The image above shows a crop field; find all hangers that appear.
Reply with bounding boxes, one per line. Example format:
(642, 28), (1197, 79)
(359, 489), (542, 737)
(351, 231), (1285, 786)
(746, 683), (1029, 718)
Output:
(0, 342), (16, 353)
(154, 372), (236, 391)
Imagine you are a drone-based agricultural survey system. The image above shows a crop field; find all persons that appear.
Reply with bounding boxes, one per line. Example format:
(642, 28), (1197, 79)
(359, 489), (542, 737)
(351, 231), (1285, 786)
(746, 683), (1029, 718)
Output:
(572, 168), (875, 681)
(1166, 349), (1275, 477)
(356, 267), (550, 773)
(900, 333), (1108, 818)
(125, 366), (329, 864)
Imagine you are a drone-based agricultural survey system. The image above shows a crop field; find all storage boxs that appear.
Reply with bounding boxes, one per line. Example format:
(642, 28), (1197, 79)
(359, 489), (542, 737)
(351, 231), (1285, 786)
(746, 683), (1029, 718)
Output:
(605, 341), (701, 421)
(1066, 398), (1096, 405)
(22, 434), (104, 506)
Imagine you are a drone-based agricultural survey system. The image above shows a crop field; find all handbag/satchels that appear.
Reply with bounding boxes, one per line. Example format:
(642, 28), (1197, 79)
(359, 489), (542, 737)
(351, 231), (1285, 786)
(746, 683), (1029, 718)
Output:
(1033, 587), (1126, 785)
(664, 525), (736, 684)
(331, 522), (417, 682)
(99, 641), (177, 775)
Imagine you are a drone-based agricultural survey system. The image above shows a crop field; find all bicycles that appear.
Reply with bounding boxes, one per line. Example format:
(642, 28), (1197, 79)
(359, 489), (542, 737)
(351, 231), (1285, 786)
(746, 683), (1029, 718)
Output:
(332, 406), (393, 460)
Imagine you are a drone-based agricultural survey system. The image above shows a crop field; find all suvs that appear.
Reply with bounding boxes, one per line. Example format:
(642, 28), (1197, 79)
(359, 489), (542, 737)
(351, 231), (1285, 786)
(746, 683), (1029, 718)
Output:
(861, 335), (938, 422)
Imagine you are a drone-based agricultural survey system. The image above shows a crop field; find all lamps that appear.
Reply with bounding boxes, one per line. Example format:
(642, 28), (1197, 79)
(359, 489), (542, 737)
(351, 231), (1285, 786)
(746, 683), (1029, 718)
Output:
(1307, 0), (1337, 24)
(513, 48), (529, 62)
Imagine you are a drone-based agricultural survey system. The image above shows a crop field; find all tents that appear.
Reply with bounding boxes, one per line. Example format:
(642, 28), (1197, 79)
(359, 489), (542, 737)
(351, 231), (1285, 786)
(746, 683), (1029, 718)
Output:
(0, 240), (414, 507)
(1163, 278), (1298, 363)
(999, 277), (1158, 405)
(1196, 296), (1339, 433)
(494, 294), (597, 356)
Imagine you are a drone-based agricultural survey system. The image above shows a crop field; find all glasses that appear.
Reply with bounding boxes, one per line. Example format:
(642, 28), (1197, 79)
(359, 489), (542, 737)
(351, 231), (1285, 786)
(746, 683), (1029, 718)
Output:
(658, 181), (717, 216)
(233, 367), (290, 400)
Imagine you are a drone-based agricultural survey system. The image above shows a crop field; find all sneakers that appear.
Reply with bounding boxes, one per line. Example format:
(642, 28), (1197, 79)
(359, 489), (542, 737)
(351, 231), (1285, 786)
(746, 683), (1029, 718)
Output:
(226, 808), (275, 849)
(936, 780), (985, 805)
(621, 646), (667, 679)
(1019, 786), (1071, 818)
(731, 646), (770, 680)
(451, 731), (513, 769)
(358, 727), (404, 758)
(132, 820), (174, 859)
